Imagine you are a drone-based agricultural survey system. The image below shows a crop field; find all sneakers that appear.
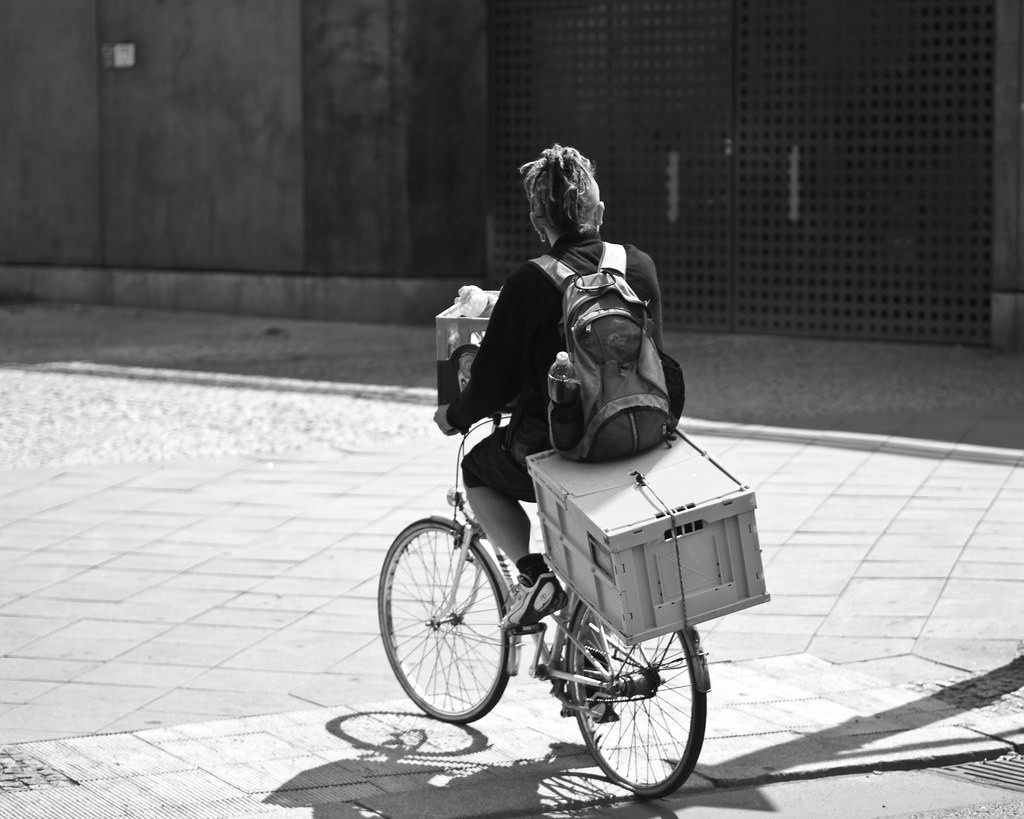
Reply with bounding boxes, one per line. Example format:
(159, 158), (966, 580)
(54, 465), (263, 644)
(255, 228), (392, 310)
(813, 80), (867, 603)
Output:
(563, 680), (612, 717)
(498, 574), (567, 630)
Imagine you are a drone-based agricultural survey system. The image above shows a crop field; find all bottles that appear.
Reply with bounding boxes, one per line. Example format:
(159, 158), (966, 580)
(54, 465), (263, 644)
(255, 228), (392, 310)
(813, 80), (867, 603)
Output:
(549, 350), (579, 408)
(454, 284), (499, 320)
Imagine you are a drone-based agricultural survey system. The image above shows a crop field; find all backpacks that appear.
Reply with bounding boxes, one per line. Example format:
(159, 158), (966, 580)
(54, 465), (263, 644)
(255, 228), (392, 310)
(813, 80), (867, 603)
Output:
(526, 242), (685, 461)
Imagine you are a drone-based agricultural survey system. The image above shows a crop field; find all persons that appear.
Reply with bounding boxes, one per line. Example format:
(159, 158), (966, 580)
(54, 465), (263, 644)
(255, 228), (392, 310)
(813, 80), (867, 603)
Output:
(431, 139), (670, 722)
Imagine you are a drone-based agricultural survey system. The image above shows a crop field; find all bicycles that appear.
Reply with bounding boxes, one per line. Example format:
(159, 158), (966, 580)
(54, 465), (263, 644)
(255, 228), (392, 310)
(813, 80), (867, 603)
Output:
(376, 402), (713, 802)
